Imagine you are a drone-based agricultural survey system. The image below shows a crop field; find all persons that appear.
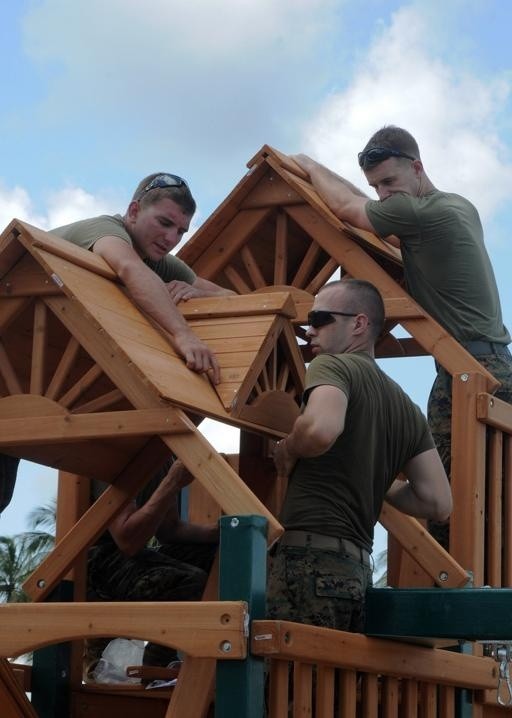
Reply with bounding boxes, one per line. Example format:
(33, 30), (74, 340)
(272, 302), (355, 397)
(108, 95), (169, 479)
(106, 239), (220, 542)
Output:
(1, 169), (239, 509)
(264, 276), (455, 718)
(86, 455), (222, 684)
(288, 124), (511, 551)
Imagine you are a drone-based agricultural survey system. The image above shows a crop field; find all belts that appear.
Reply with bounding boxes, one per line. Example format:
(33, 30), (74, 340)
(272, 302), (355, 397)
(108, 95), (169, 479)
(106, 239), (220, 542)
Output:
(275, 530), (369, 563)
(467, 341), (511, 355)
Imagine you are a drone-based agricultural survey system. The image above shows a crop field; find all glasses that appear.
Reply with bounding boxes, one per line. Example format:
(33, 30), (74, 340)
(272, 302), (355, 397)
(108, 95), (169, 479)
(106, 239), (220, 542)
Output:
(136, 174), (189, 200)
(358, 148), (417, 166)
(308, 311), (370, 328)
